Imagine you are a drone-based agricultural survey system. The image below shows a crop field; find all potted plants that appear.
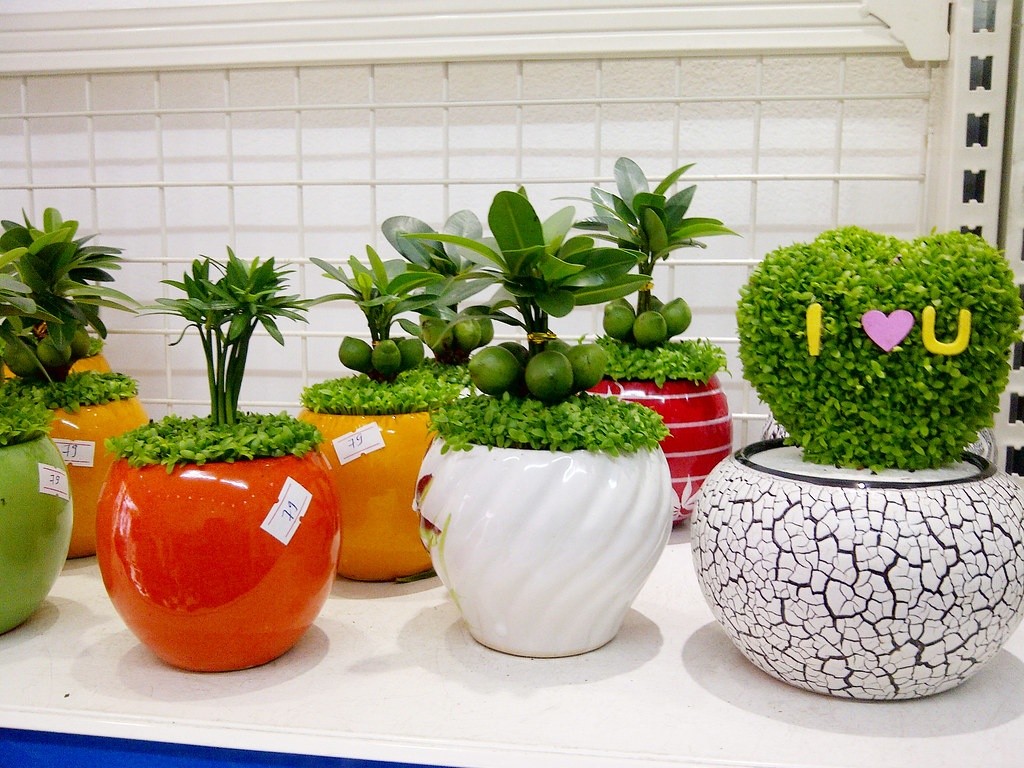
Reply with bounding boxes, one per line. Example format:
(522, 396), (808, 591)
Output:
(0, 207), (1024, 703)
